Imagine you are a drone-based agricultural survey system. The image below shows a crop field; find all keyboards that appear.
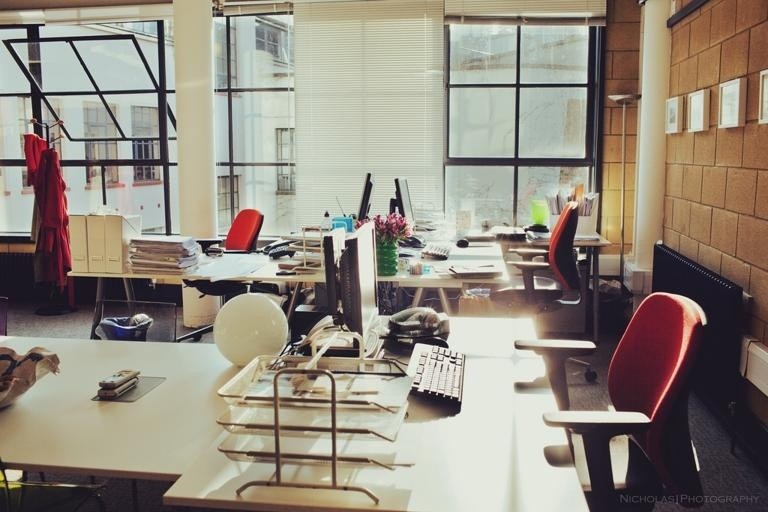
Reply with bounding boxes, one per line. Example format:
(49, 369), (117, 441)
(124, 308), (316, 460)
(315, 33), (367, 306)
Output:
(269, 245), (296, 259)
(421, 242), (452, 259)
(405, 342), (466, 409)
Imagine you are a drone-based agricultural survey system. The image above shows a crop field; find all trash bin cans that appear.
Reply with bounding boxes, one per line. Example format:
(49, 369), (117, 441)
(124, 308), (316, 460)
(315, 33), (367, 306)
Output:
(593, 281), (634, 340)
(99, 317), (153, 341)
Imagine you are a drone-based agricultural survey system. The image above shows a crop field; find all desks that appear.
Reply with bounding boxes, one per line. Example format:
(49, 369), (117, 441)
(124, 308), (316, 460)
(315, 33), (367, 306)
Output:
(0, 226), (611, 509)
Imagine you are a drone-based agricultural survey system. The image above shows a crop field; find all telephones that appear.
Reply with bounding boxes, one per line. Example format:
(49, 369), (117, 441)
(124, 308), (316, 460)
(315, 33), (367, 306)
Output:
(262, 240), (297, 259)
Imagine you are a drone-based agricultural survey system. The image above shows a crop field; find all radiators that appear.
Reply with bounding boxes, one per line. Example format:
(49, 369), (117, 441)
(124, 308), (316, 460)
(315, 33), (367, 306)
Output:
(651, 243), (744, 453)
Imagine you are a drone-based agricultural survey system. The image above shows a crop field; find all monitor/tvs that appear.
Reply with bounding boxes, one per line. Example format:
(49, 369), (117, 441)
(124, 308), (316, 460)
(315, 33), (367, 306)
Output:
(390, 178), (414, 219)
(358, 173), (373, 220)
(340, 221), (381, 349)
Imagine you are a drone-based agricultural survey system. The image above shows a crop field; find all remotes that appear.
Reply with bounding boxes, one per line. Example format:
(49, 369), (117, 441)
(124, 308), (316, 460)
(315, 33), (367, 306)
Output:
(97, 375), (139, 399)
(99, 368), (140, 388)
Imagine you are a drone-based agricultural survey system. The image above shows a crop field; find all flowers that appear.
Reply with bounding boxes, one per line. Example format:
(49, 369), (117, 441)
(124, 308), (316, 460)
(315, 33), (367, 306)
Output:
(354, 214), (413, 246)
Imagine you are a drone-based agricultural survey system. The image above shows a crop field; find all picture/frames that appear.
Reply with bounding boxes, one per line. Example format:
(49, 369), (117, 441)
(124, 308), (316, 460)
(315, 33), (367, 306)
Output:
(665, 69), (768, 135)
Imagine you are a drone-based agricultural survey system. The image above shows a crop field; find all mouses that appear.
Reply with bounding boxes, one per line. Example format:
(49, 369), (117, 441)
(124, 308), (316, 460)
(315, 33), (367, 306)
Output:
(457, 238), (468, 247)
(423, 336), (449, 347)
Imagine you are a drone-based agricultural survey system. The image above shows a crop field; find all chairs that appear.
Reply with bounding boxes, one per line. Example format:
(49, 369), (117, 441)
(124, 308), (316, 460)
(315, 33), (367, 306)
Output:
(514, 291), (709, 511)
(182, 210), (264, 342)
(488, 201), (598, 381)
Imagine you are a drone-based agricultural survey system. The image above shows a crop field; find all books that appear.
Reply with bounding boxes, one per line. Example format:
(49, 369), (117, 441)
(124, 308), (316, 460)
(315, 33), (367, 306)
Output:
(449, 267), (503, 278)
(545, 185), (600, 216)
(129, 235), (202, 274)
